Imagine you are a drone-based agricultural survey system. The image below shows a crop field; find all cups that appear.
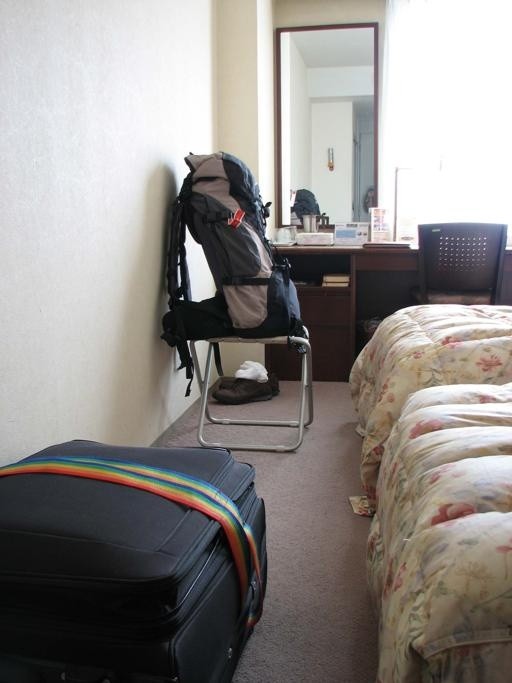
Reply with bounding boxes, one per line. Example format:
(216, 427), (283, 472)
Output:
(276, 227), (294, 244)
(302, 213), (321, 232)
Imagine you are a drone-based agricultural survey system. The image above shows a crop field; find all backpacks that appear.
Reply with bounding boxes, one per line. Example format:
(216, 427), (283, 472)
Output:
(166, 151), (303, 335)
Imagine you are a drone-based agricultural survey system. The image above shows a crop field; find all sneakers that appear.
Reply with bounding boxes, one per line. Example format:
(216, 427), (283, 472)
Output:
(212, 373), (280, 405)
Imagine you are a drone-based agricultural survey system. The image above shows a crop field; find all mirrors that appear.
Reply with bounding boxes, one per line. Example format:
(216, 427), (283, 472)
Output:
(275, 22), (379, 229)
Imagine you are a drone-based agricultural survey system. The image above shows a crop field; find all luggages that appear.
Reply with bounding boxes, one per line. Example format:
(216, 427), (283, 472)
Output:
(0, 439), (267, 682)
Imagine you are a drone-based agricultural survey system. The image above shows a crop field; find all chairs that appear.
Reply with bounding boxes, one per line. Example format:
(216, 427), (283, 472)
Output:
(408, 224), (509, 311)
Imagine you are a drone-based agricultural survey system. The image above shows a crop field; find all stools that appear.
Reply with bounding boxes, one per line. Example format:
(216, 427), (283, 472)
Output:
(189, 326), (314, 453)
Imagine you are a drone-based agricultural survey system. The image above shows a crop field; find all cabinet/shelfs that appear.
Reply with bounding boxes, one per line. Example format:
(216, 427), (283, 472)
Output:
(266, 247), (511, 382)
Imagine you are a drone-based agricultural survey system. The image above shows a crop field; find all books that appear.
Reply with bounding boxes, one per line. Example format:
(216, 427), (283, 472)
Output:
(321, 281), (349, 287)
(322, 272), (351, 282)
(361, 241), (410, 248)
